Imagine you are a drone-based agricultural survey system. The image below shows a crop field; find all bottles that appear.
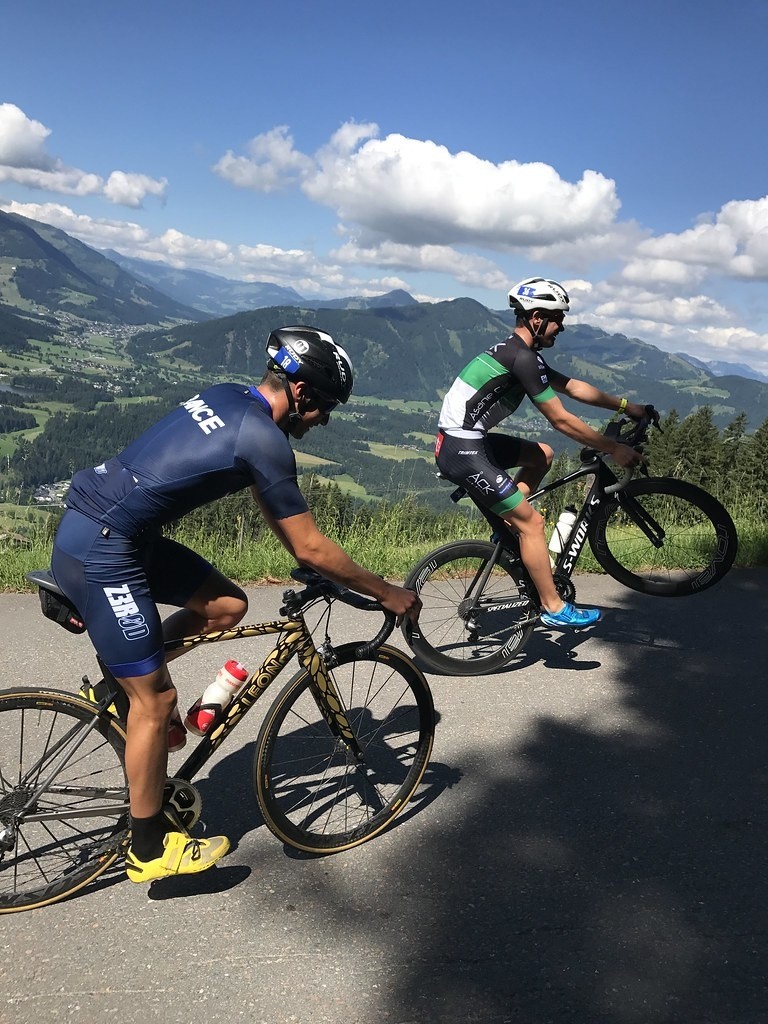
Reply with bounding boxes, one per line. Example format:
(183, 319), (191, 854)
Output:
(548, 504), (578, 553)
(184, 660), (250, 736)
(167, 705), (187, 752)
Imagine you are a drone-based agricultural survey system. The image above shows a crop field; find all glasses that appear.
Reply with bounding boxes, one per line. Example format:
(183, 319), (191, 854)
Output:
(308, 388), (338, 414)
(539, 314), (564, 323)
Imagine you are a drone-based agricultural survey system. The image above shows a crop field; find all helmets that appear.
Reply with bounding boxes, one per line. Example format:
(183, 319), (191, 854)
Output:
(509, 280), (570, 314)
(266, 325), (353, 404)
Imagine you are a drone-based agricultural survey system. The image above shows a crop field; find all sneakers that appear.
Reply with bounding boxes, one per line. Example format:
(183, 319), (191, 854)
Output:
(540, 601), (601, 627)
(78, 675), (121, 717)
(126, 831), (230, 883)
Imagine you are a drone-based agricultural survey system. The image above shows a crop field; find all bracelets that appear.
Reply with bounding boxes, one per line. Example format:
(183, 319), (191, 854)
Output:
(617, 398), (628, 414)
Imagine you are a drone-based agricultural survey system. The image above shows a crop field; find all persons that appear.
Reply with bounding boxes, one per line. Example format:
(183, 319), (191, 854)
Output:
(435, 277), (661, 627)
(51, 325), (422, 882)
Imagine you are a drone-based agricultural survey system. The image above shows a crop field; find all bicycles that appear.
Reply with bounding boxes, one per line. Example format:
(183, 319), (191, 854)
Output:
(398, 404), (737, 676)
(0, 565), (437, 914)
